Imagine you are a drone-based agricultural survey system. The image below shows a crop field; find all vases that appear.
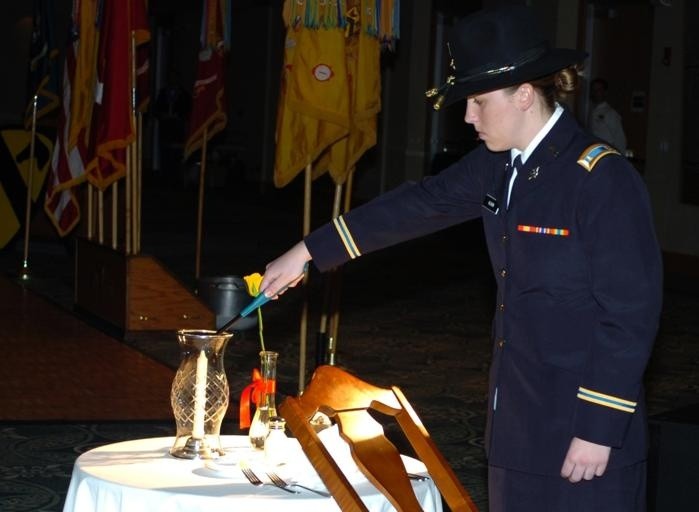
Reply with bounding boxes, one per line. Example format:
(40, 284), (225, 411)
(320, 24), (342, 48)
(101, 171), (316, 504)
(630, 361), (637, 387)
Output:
(248, 350), (286, 453)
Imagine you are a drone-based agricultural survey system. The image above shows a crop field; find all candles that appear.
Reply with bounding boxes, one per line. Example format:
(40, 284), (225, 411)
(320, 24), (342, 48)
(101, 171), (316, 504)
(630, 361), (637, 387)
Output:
(191, 350), (208, 440)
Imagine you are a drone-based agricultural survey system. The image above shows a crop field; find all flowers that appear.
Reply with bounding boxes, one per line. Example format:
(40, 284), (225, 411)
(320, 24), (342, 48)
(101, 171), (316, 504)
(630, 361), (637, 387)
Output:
(242, 273), (276, 418)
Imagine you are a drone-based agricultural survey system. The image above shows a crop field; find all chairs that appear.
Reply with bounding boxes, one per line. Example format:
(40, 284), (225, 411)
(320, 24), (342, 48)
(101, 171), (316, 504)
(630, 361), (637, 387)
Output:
(277, 363), (482, 510)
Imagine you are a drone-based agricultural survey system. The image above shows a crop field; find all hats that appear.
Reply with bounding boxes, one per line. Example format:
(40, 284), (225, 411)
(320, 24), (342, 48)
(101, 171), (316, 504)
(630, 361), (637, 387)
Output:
(424, 8), (589, 112)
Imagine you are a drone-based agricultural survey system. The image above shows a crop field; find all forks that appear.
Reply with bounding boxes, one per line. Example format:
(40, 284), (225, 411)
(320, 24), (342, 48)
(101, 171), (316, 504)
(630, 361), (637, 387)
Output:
(240, 466), (302, 495)
(267, 471), (334, 499)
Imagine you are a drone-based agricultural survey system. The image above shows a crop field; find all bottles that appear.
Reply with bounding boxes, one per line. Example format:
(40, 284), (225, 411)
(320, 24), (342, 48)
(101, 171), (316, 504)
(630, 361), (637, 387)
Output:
(262, 416), (292, 465)
(249, 349), (280, 451)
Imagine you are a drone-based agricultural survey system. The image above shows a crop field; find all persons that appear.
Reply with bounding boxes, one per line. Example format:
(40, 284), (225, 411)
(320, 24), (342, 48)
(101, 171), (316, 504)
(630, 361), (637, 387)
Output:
(586, 74), (628, 154)
(258, 46), (664, 512)
